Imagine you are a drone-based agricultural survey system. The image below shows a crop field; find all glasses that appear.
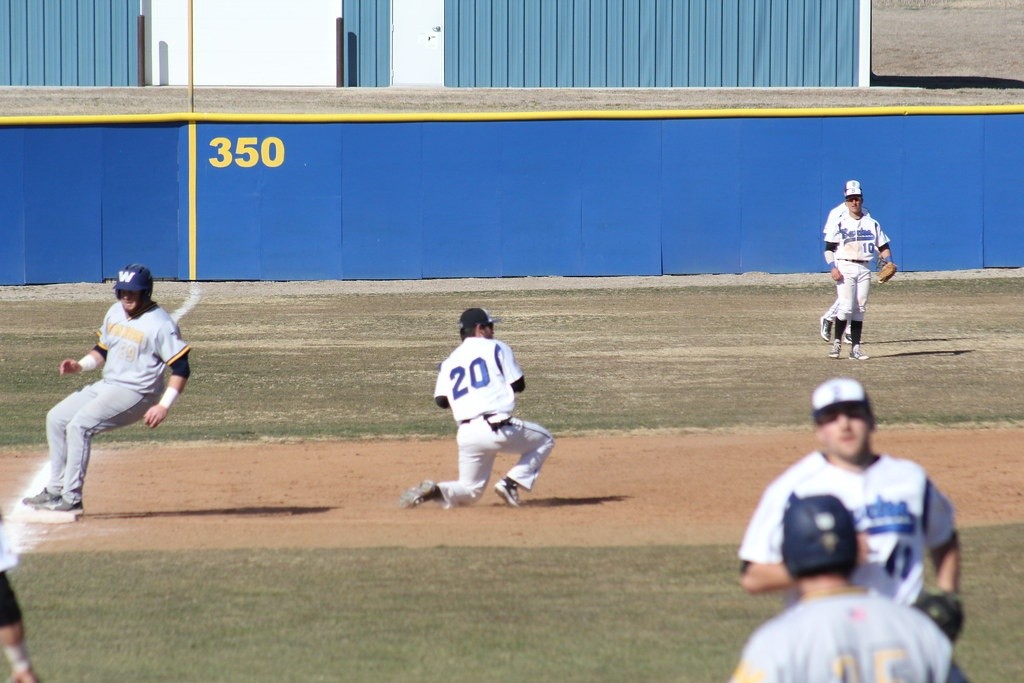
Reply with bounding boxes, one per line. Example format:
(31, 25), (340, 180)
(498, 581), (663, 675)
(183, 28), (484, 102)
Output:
(847, 197), (859, 202)
(813, 402), (867, 425)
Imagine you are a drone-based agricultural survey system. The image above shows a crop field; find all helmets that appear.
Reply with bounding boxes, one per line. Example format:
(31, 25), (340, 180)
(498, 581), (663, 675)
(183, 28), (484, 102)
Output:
(112, 264), (153, 291)
(782, 496), (856, 575)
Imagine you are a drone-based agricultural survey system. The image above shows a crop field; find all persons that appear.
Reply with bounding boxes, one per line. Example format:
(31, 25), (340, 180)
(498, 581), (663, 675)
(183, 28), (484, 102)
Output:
(0, 513), (40, 683)
(726, 496), (968, 682)
(740, 378), (964, 639)
(22, 264), (190, 513)
(401, 308), (555, 511)
(820, 180), (896, 359)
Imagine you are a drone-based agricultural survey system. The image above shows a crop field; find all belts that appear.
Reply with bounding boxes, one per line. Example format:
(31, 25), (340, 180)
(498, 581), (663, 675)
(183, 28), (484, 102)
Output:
(460, 413), (495, 424)
(845, 259), (869, 263)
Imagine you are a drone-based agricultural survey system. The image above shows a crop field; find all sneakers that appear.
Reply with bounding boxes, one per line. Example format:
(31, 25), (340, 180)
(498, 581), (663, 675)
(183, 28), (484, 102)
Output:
(849, 350), (869, 359)
(494, 480), (520, 508)
(399, 480), (437, 509)
(821, 316), (833, 342)
(829, 342), (842, 358)
(36, 497), (85, 515)
(844, 335), (864, 344)
(22, 487), (61, 505)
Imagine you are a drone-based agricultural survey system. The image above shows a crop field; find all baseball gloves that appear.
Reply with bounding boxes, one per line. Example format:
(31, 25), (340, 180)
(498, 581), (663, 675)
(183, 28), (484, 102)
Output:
(911, 588), (965, 643)
(877, 262), (897, 284)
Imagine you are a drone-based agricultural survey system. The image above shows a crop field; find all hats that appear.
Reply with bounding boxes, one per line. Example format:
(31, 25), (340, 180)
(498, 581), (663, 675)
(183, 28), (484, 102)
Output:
(812, 379), (865, 410)
(845, 181), (861, 189)
(845, 188), (862, 197)
(459, 309), (496, 329)
